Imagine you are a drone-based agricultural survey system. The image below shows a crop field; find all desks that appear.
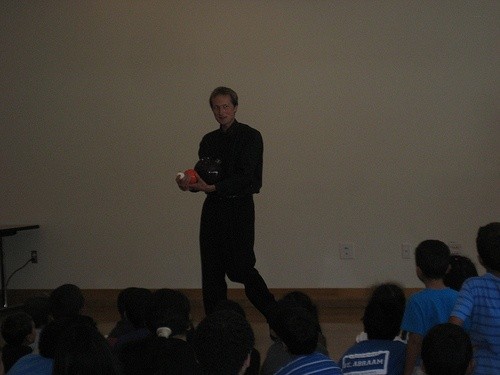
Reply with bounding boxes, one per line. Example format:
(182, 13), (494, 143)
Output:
(0, 224), (40, 309)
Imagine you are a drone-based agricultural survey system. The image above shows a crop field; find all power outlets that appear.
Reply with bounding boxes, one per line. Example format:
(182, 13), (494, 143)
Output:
(30, 251), (38, 264)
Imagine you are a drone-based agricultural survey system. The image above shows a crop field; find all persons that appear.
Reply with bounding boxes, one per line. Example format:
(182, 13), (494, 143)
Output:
(338, 293), (420, 375)
(195, 310), (256, 375)
(443, 255), (478, 291)
(400, 240), (472, 375)
(273, 306), (344, 375)
(8, 316), (67, 375)
(261, 291), (329, 375)
(0, 313), (37, 375)
(210, 299), (261, 375)
(109, 287), (196, 375)
(421, 323), (474, 375)
(176, 86), (279, 326)
(48, 283), (84, 322)
(449, 222), (500, 375)
(356, 283), (409, 344)
(51, 331), (116, 375)
